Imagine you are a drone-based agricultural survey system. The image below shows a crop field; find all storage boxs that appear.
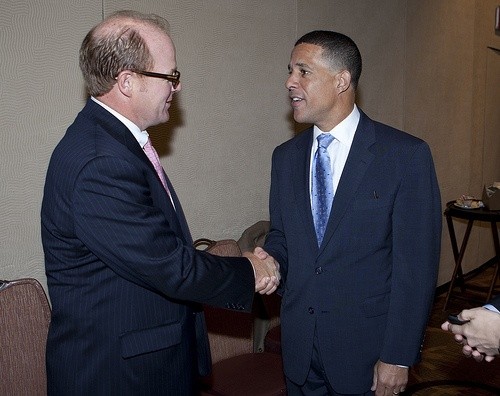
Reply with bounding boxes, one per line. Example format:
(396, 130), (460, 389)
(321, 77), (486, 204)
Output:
(483, 184), (500, 211)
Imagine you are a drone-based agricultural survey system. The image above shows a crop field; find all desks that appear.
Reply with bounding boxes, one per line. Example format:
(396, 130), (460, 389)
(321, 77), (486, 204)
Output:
(442, 199), (500, 314)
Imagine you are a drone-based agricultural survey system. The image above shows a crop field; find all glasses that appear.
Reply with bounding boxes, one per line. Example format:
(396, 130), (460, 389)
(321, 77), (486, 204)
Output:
(114, 69), (181, 90)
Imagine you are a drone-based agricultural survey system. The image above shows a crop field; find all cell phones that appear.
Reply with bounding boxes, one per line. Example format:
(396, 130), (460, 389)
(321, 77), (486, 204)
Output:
(446, 312), (470, 326)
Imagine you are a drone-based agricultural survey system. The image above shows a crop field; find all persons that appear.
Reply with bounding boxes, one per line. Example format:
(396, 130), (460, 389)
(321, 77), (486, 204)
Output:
(441, 298), (500, 362)
(41, 12), (281, 396)
(249, 29), (443, 396)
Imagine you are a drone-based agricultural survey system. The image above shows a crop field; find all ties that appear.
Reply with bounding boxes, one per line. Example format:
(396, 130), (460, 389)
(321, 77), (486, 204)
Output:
(144, 137), (169, 198)
(311, 132), (335, 247)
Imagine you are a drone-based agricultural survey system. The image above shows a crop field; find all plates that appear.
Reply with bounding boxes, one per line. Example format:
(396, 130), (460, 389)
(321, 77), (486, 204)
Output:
(454, 203), (480, 209)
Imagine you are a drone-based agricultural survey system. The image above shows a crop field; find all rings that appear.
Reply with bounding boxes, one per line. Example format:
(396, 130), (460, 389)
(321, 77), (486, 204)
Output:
(393, 391), (399, 396)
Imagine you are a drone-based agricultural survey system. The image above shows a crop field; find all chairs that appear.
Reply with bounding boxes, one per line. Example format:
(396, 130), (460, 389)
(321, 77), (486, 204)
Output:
(0, 278), (52, 396)
(192, 238), (287, 396)
(236, 219), (286, 354)
